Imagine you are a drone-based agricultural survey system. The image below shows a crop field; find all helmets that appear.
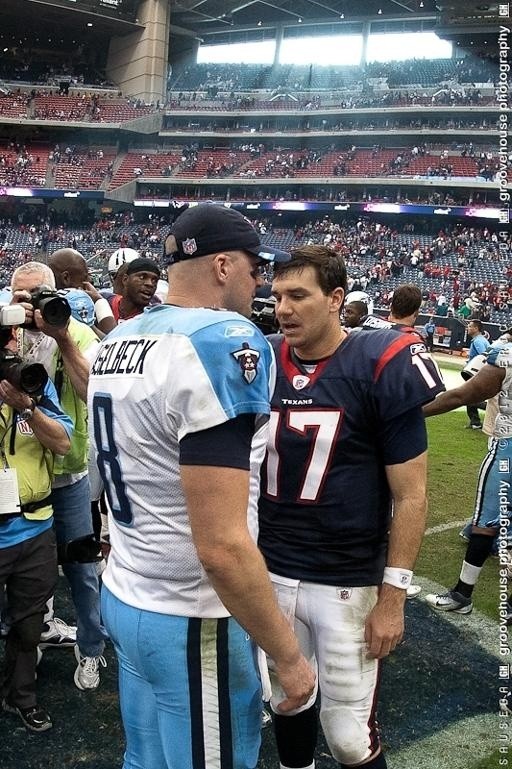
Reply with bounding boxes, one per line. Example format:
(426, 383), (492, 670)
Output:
(342, 291), (373, 315)
(58, 288), (95, 326)
(107, 247), (141, 283)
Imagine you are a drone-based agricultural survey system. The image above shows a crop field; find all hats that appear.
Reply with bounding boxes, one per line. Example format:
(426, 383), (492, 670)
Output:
(163, 197), (291, 266)
(127, 258), (160, 275)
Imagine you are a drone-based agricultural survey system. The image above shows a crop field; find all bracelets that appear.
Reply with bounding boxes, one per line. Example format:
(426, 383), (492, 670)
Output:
(381, 565), (414, 591)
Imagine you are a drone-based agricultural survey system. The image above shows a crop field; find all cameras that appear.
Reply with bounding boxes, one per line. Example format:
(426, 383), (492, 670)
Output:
(0, 347), (49, 396)
(250, 296), (280, 336)
(18, 285), (72, 332)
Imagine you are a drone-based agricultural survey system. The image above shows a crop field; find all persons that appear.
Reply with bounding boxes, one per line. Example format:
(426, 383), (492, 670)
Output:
(0, 260), (108, 693)
(86, 189), (317, 768)
(422, 324), (512, 626)
(0, 50), (512, 430)
(36, 429), (116, 648)
(254, 245), (434, 768)
(0, 326), (77, 732)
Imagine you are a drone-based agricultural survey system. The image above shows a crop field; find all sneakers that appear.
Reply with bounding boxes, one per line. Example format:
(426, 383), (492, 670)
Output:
(1, 695), (53, 731)
(425, 592), (473, 615)
(405, 585), (420, 598)
(39, 619), (77, 649)
(261, 708), (272, 729)
(73, 645), (107, 691)
